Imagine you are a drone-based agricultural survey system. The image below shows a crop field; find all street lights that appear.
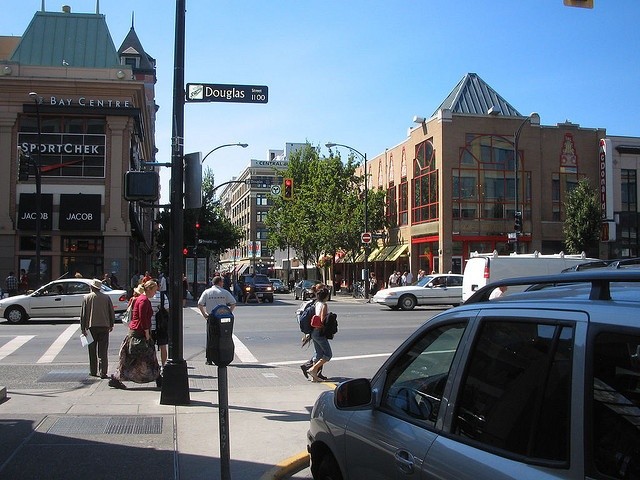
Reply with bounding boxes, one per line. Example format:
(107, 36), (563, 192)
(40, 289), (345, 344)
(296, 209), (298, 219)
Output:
(192, 142), (252, 301)
(513, 112), (540, 251)
(17, 91), (41, 289)
(326, 143), (370, 299)
(203, 179), (253, 206)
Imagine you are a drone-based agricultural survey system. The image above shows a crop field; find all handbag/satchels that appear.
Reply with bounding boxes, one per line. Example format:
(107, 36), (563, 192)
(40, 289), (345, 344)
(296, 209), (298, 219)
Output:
(311, 306), (326, 329)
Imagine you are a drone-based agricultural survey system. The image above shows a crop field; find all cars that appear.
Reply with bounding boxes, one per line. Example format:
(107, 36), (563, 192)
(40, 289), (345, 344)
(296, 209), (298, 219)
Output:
(373, 274), (464, 310)
(294, 280), (332, 300)
(524, 259), (640, 291)
(239, 273), (274, 303)
(0, 279), (128, 324)
(270, 278), (289, 294)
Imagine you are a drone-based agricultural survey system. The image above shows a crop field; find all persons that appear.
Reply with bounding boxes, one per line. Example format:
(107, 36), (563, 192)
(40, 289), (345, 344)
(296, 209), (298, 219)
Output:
(78, 277), (116, 380)
(389, 270), (398, 287)
(142, 270), (150, 284)
(127, 284), (144, 321)
(396, 273), (402, 287)
(55, 286), (63, 296)
(301, 284), (328, 380)
(244, 274), (263, 306)
(489, 286), (508, 300)
(108, 280), (168, 389)
(159, 272), (166, 306)
(231, 273), (242, 304)
(156, 270), (161, 274)
(416, 268), (421, 282)
(404, 271), (409, 286)
(20, 268), (29, 295)
(74, 273), (82, 278)
(130, 269), (139, 292)
(136, 274), (144, 287)
(421, 270), (426, 281)
(198, 273), (237, 368)
(102, 274), (110, 287)
(300, 289), (332, 381)
(368, 272), (377, 303)
(110, 271), (120, 290)
(224, 270), (233, 293)
(4, 271), (18, 297)
(183, 273), (188, 308)
(434, 278), (445, 287)
(148, 278), (169, 368)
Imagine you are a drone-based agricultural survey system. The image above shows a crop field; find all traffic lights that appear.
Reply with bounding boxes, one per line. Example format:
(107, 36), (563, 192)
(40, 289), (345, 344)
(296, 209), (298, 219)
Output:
(193, 221), (202, 231)
(285, 179), (294, 195)
(514, 211), (522, 233)
(183, 248), (189, 257)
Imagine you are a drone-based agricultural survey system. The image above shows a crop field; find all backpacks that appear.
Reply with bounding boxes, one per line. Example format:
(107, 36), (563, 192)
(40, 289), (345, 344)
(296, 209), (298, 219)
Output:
(300, 301), (315, 334)
(156, 291), (170, 347)
(296, 300), (311, 330)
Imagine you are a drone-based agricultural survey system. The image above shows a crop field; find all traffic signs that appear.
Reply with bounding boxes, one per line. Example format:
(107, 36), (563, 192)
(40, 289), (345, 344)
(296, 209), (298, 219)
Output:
(271, 185), (281, 196)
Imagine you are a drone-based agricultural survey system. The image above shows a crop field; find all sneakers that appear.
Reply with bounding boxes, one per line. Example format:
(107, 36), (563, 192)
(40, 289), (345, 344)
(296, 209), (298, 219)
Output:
(100, 374), (107, 379)
(307, 368), (321, 381)
(88, 372), (97, 376)
(317, 373), (327, 379)
(205, 359), (213, 365)
(108, 375), (127, 389)
(301, 363), (308, 379)
(157, 377), (163, 390)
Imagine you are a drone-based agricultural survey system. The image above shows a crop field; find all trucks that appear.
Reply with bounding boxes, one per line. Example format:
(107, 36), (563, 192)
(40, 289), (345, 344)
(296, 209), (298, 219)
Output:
(460, 250), (608, 303)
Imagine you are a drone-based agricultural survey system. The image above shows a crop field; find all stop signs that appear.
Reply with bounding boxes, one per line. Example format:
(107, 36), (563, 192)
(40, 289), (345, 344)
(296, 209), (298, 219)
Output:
(361, 233), (372, 244)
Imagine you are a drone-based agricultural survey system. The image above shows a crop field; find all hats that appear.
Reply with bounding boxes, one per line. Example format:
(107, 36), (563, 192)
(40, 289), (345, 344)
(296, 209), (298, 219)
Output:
(89, 279), (106, 290)
(133, 283), (145, 295)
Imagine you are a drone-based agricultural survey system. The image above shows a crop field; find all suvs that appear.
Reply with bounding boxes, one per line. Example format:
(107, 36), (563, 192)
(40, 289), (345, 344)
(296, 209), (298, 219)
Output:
(307, 262), (640, 479)
